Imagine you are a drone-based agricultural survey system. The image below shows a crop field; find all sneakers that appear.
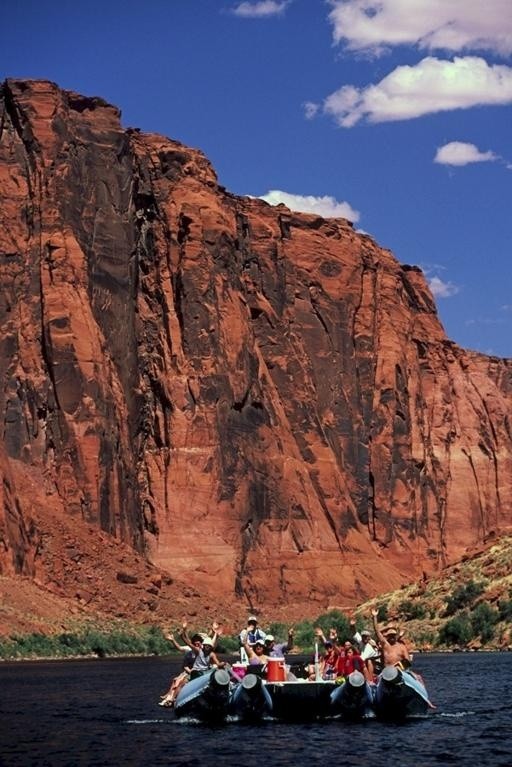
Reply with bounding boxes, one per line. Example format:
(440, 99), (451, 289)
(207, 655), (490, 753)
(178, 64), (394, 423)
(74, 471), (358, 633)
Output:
(158, 695), (175, 707)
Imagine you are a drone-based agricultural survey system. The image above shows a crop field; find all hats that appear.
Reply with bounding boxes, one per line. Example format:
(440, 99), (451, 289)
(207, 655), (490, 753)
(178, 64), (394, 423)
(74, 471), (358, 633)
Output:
(256, 634), (274, 645)
(202, 636), (214, 647)
(324, 640), (333, 647)
(361, 630), (373, 636)
(247, 616), (257, 622)
(386, 629), (397, 635)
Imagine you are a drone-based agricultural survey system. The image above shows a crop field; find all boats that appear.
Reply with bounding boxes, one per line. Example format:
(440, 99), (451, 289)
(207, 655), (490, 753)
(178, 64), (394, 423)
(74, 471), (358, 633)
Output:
(171, 661), (431, 722)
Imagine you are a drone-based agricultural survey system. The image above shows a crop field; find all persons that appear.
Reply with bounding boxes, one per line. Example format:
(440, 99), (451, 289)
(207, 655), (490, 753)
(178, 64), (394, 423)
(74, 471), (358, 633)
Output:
(158, 621), (220, 705)
(239, 615), (266, 665)
(243, 625), (271, 679)
(159, 628), (209, 700)
(264, 628), (295, 659)
(302, 608), (410, 683)
(182, 621), (219, 643)
(164, 625), (237, 708)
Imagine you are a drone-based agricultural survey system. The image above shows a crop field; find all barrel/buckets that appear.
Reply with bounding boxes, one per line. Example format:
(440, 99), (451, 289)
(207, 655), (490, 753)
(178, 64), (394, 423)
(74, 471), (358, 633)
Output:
(266, 657), (286, 681)
